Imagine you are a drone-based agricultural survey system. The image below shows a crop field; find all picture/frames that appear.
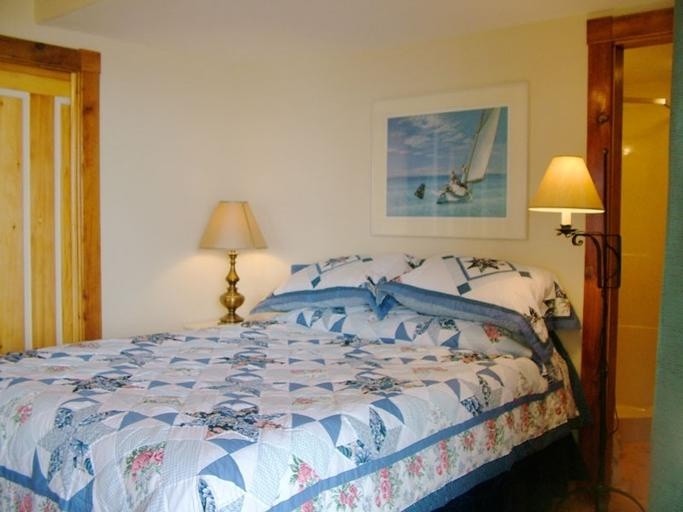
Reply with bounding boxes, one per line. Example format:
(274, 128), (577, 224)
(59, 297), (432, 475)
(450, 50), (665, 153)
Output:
(370, 80), (528, 241)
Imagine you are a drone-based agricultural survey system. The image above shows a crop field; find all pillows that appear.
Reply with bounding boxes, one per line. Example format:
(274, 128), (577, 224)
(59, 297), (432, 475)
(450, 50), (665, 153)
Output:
(250, 255), (418, 320)
(381, 252), (581, 358)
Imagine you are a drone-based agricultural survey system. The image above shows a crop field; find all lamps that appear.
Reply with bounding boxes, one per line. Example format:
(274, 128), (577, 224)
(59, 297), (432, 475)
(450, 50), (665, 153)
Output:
(202, 202), (265, 327)
(527, 153), (622, 289)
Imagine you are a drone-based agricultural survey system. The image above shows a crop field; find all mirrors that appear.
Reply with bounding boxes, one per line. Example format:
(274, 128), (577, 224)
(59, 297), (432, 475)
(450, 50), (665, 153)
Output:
(577, 3), (672, 511)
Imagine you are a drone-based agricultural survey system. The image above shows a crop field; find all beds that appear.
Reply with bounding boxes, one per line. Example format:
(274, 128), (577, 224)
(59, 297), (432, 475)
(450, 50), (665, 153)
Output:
(0, 252), (587, 511)
